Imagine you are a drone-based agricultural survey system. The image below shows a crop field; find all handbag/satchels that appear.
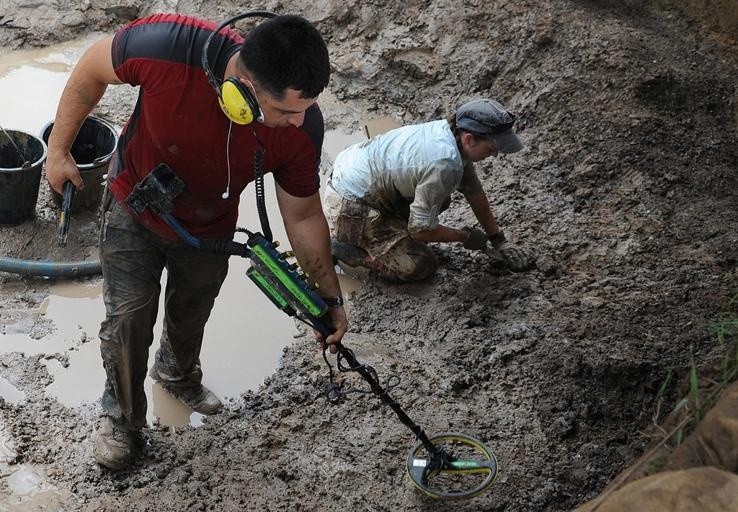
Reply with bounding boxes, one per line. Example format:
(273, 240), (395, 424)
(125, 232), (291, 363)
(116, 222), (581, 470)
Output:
(316, 293), (343, 305)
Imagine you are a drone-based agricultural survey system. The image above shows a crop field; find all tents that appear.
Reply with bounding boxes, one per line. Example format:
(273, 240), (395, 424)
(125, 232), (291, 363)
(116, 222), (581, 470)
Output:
(201, 11), (281, 127)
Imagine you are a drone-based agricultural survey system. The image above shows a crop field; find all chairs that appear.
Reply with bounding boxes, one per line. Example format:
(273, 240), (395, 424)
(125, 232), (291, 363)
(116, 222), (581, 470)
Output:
(92, 414), (136, 470)
(149, 366), (222, 416)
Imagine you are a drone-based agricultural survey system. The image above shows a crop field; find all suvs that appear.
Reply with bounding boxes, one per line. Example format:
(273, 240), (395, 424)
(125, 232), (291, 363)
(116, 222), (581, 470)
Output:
(0, 127), (49, 226)
(38, 117), (119, 214)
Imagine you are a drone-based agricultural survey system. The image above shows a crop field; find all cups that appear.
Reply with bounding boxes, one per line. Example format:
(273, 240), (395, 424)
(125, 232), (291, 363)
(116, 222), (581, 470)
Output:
(464, 111), (518, 133)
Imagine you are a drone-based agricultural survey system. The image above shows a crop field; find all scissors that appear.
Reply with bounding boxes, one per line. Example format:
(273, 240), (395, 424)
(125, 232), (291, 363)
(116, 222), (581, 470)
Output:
(462, 225), (505, 261)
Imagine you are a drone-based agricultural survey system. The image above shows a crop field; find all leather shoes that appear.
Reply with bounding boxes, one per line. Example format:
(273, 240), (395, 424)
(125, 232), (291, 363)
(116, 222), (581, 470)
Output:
(491, 230), (527, 272)
(460, 224), (487, 251)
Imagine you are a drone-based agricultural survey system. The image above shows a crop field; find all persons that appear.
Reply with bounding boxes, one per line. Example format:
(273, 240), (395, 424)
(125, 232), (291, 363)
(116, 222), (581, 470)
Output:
(566, 378), (736, 510)
(324, 97), (533, 286)
(45, 13), (351, 468)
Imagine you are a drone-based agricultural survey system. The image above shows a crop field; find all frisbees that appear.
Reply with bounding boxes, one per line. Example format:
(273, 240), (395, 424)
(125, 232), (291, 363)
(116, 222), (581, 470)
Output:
(220, 189), (231, 200)
(257, 110), (265, 125)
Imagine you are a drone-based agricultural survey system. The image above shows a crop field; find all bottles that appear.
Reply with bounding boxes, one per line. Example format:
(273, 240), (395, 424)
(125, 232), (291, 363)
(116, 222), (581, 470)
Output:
(455, 97), (526, 155)
